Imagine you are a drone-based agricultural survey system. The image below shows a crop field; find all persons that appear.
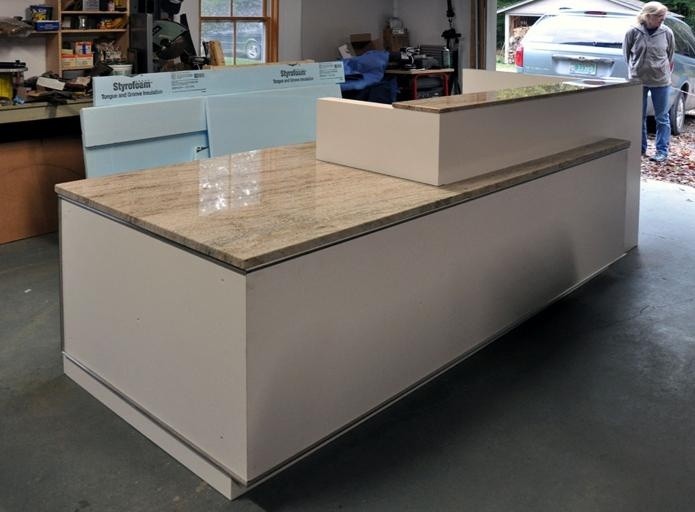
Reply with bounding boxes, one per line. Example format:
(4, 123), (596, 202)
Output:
(620, 2), (675, 165)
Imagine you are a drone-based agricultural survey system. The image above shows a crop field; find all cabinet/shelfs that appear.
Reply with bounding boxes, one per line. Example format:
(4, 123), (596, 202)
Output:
(45, 0), (130, 81)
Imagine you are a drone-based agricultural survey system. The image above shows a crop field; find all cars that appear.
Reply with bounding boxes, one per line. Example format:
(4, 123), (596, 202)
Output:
(206, 23), (262, 60)
(513, 7), (695, 135)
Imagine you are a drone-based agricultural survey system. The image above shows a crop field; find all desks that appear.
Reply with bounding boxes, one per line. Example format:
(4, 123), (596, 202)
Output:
(385, 68), (455, 99)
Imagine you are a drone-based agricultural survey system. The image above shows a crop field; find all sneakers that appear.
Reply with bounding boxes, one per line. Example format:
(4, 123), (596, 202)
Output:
(649, 153), (667, 162)
